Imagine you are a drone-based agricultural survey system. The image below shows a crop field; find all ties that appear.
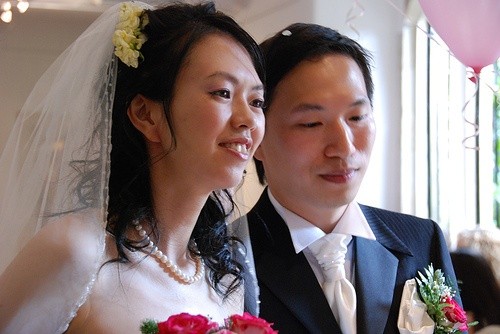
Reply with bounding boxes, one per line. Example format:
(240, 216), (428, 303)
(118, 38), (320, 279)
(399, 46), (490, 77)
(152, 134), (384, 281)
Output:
(307, 234), (357, 334)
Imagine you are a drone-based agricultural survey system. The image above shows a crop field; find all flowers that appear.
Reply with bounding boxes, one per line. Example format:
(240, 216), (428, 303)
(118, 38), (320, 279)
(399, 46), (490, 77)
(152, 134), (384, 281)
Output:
(111, 1), (150, 69)
(138, 312), (277, 334)
(415, 262), (479, 333)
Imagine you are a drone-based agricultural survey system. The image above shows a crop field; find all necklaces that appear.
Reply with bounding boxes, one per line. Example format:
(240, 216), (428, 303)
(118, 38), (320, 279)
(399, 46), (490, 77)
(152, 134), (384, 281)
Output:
(128, 214), (203, 284)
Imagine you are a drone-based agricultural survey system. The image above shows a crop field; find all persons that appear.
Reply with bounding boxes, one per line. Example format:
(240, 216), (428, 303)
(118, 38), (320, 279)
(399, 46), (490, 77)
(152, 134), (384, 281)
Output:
(224, 23), (467, 334)
(0, 0), (278, 333)
(448, 251), (500, 334)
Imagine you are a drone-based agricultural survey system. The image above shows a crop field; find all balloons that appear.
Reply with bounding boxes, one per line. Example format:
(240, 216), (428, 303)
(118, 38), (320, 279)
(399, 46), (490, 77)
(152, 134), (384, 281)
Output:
(417, 0), (500, 84)
